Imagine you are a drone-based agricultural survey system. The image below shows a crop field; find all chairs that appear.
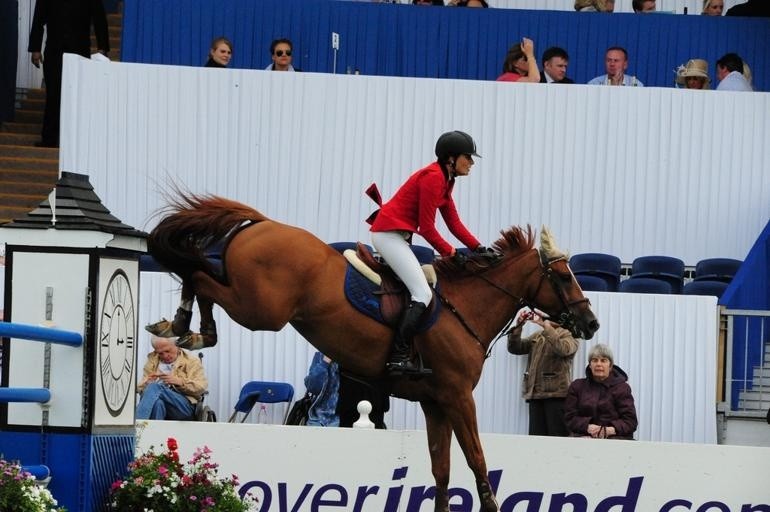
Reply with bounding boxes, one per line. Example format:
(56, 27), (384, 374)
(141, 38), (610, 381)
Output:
(229, 392), (260, 423)
(232, 381), (294, 425)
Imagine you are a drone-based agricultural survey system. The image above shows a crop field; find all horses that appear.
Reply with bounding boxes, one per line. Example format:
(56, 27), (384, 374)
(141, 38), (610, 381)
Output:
(142, 168), (602, 512)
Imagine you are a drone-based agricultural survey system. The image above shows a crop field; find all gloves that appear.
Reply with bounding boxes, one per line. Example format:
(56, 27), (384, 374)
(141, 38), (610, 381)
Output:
(451, 250), (473, 276)
(479, 244), (499, 265)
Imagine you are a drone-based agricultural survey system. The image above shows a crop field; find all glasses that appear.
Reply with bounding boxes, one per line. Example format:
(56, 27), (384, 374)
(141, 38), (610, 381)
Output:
(275, 49), (292, 57)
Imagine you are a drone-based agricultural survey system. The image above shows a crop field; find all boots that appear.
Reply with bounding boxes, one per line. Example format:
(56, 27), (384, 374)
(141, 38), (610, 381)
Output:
(384, 301), (434, 374)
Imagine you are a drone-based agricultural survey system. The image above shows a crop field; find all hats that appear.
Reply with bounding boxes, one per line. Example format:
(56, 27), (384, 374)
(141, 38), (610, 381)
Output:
(676, 59), (711, 84)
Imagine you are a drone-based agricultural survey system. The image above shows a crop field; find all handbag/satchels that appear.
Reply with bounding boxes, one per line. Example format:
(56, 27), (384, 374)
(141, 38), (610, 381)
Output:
(286, 396), (311, 425)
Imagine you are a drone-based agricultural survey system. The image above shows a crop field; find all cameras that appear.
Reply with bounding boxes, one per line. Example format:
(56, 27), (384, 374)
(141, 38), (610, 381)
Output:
(524, 312), (534, 320)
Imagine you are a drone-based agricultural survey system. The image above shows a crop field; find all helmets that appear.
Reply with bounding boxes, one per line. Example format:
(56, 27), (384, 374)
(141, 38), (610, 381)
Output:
(435, 131), (483, 159)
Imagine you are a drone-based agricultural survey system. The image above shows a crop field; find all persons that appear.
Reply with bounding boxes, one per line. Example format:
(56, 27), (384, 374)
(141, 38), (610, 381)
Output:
(364, 130), (497, 375)
(135, 334), (209, 420)
(304, 350), (340, 427)
(573, 0), (769, 25)
(265, 38), (302, 72)
(203, 36), (233, 69)
(28, 1), (111, 147)
(495, 37), (769, 92)
(506, 308), (580, 436)
(563, 342), (639, 440)
(384, 1), (487, 9)
